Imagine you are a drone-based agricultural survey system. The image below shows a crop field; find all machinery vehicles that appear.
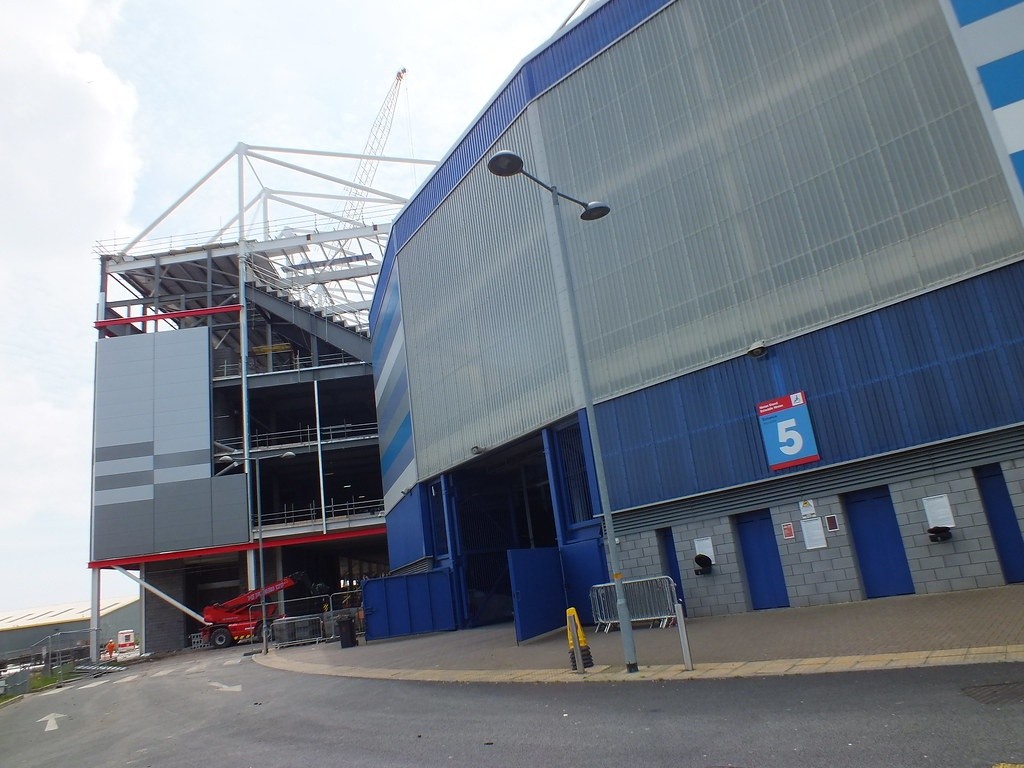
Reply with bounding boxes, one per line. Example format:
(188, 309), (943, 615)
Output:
(199, 570), (329, 650)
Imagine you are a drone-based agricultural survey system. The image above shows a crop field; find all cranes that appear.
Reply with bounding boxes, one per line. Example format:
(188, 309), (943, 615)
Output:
(300, 65), (408, 321)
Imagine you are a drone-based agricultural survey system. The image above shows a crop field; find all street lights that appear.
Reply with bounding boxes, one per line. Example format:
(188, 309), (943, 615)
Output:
(218, 451), (298, 657)
(486, 149), (640, 674)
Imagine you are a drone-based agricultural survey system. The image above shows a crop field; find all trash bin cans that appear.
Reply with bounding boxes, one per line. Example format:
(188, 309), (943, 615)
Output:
(337, 617), (360, 648)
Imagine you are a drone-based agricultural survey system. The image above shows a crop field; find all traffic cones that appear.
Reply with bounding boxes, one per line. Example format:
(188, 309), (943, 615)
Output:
(565, 606), (594, 670)
